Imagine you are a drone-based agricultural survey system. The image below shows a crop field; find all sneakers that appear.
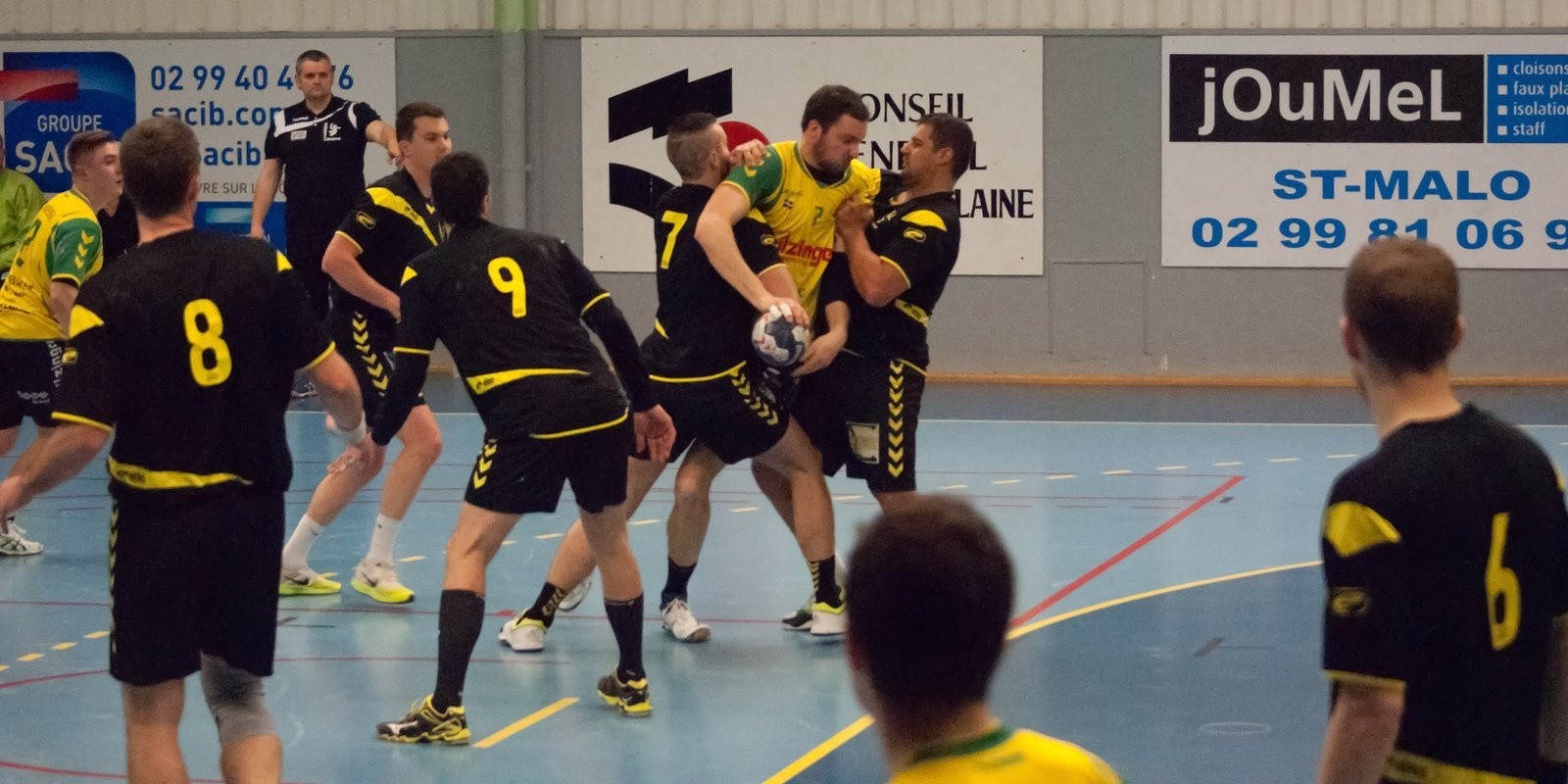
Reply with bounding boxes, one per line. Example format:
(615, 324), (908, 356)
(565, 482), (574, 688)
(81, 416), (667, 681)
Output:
(811, 599), (848, 633)
(1, 523), (43, 555)
(597, 669), (652, 711)
(659, 597), (711, 642)
(782, 595), (819, 627)
(280, 564), (341, 596)
(498, 607), (546, 651)
(558, 575), (595, 610)
(375, 694), (469, 744)
(350, 560), (414, 603)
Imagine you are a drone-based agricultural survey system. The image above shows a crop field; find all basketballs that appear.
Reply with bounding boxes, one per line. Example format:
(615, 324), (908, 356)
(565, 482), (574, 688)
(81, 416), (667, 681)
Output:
(752, 312), (814, 366)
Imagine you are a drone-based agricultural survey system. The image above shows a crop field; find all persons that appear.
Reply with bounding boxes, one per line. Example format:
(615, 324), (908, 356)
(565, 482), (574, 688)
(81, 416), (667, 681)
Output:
(249, 48), (399, 318)
(0, 114), (368, 784)
(1316, 235), (1568, 784)
(490, 86), (974, 650)
(844, 494), (1124, 784)
(271, 103), (474, 608)
(362, 154), (680, 747)
